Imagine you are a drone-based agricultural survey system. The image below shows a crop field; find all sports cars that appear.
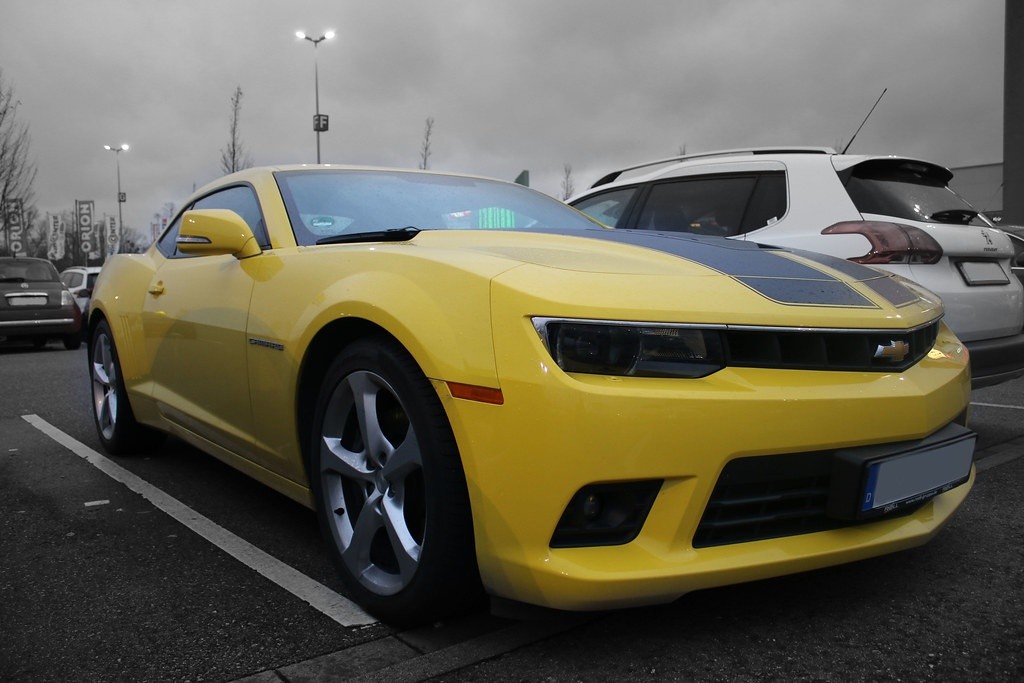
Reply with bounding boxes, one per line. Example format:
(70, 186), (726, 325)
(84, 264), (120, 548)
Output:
(85, 163), (977, 625)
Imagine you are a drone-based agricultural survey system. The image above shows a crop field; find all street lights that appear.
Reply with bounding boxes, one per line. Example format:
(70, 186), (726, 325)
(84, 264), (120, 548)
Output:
(295, 28), (336, 163)
(104, 144), (131, 251)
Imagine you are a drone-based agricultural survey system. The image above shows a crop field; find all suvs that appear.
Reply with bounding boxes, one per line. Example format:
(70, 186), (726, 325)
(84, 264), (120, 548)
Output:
(562, 146), (1022, 392)
(57, 266), (102, 296)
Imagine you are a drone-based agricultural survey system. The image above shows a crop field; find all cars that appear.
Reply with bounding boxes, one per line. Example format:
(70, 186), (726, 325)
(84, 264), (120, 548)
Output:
(0, 256), (87, 352)
